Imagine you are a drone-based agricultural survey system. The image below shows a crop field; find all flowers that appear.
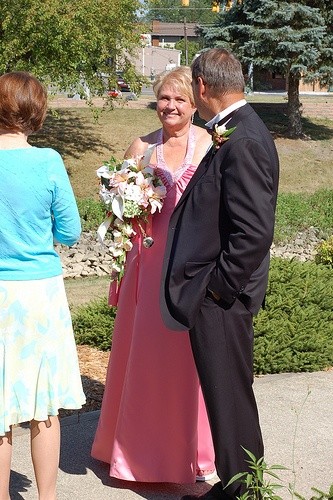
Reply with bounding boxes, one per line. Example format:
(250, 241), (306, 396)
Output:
(95, 153), (167, 294)
(209, 116), (237, 158)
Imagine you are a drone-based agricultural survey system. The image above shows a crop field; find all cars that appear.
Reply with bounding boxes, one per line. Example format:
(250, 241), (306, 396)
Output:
(108, 71), (143, 93)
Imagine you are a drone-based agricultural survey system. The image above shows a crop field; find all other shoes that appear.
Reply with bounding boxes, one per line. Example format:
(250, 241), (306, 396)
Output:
(182, 482), (232, 500)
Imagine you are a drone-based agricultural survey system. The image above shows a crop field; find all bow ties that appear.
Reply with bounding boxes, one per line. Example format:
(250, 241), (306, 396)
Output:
(206, 108), (241, 140)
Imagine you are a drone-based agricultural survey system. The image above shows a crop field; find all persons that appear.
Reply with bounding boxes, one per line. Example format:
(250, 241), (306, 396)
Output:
(0, 71), (85, 500)
(90, 46), (279, 500)
